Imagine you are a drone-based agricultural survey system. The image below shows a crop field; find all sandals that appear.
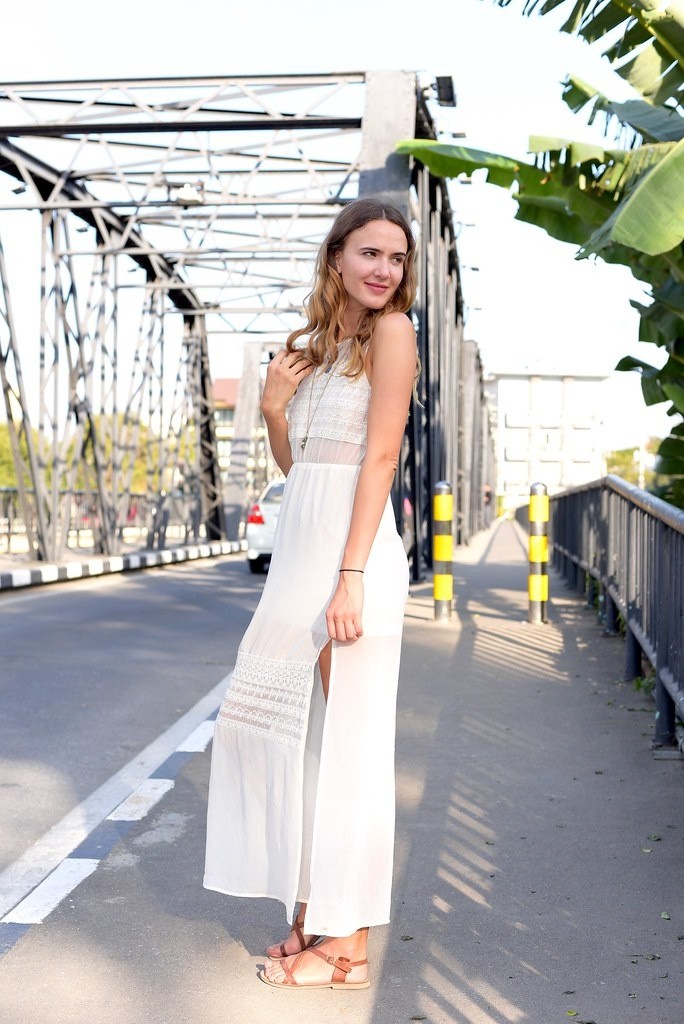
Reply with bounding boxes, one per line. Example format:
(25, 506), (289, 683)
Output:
(258, 943), (370, 989)
(266, 915), (320, 959)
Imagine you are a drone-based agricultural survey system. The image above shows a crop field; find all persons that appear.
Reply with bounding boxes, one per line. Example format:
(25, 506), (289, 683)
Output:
(202, 197), (417, 990)
(483, 484), (491, 528)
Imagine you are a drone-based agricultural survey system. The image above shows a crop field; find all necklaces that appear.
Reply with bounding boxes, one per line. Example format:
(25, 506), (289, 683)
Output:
(300, 334), (351, 449)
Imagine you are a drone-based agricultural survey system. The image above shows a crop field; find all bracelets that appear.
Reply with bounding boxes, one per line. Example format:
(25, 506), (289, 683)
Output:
(338, 569), (364, 574)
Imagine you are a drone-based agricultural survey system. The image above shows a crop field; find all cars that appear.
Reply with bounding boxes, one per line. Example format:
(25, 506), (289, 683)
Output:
(245, 478), (287, 574)
